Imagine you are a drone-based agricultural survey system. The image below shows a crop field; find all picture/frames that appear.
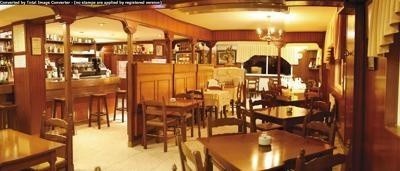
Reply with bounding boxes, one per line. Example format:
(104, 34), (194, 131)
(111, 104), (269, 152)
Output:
(217, 50), (236, 66)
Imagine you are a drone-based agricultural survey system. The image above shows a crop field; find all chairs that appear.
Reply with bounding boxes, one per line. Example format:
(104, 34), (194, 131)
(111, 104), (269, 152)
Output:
(30, 109), (74, 171)
(143, 76), (340, 171)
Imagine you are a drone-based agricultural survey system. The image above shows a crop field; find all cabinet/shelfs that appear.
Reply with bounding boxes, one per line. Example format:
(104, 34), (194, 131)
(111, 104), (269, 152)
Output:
(1, 37), (96, 79)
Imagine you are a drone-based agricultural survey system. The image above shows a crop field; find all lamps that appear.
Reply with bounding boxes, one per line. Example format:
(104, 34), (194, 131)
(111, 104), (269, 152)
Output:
(256, 15), (284, 45)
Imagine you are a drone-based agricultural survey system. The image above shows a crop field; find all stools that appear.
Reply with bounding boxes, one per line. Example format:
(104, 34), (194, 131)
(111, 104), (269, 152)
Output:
(52, 97), (75, 136)
(88, 89), (127, 129)
(0, 103), (17, 130)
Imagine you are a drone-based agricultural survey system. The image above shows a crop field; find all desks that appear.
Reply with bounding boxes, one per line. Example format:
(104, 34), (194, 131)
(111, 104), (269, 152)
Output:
(0, 128), (67, 171)
